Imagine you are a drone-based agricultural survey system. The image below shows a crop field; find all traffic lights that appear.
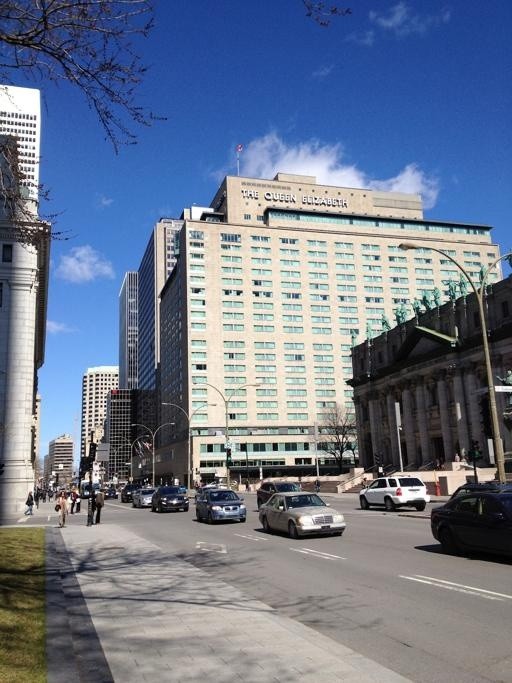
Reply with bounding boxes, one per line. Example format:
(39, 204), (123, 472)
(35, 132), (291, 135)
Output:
(375, 452), (382, 465)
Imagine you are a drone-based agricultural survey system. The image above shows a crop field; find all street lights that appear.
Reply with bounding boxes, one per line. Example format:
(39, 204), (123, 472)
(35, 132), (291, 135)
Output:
(106, 381), (262, 490)
(399, 241), (511, 483)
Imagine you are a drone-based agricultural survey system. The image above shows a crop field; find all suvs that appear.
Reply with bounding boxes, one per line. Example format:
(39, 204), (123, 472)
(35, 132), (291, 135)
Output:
(357, 475), (431, 511)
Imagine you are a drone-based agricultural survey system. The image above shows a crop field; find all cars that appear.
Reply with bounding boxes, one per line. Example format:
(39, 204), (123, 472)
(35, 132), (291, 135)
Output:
(60, 481), (346, 538)
(430, 480), (512, 556)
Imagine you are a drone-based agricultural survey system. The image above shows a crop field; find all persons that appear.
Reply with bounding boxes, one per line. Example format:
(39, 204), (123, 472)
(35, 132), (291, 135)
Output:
(246, 480), (248, 491)
(92, 495), (96, 515)
(25, 488), (81, 528)
(362, 478), (367, 489)
(315, 480), (320, 492)
(95, 489), (105, 523)
